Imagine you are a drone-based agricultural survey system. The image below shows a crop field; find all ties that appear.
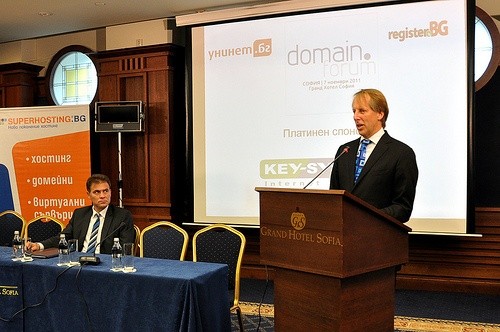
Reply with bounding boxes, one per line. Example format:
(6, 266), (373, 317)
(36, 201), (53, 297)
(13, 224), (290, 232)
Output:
(354, 138), (371, 183)
(86, 214), (101, 254)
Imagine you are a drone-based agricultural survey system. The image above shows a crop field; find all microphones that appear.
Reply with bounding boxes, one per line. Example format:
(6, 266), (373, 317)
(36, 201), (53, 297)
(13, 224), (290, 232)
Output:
(79, 222), (126, 265)
(304, 147), (351, 189)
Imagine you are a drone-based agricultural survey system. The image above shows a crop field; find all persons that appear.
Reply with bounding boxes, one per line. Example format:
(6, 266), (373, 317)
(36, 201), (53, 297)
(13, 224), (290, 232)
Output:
(26, 174), (135, 257)
(330, 88), (418, 223)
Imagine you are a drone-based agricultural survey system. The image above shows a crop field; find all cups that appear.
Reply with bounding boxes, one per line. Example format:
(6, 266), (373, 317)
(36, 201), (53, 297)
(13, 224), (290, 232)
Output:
(123, 243), (135, 271)
(67, 239), (79, 265)
(22, 237), (32, 261)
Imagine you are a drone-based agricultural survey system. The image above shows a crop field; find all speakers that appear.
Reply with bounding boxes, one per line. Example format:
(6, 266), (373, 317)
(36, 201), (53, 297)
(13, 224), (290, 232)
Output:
(95, 101), (144, 133)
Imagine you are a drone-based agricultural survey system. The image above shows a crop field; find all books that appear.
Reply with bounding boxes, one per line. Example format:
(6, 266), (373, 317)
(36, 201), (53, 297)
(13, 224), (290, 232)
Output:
(31, 247), (59, 259)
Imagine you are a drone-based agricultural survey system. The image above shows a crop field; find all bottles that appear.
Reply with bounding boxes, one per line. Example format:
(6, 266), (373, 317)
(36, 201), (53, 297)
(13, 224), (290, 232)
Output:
(13, 231), (22, 260)
(58, 234), (68, 265)
(112, 238), (123, 270)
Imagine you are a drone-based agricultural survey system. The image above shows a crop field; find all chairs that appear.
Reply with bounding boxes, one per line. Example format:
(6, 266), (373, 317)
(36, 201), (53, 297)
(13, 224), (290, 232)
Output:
(0, 210), (27, 239)
(133, 224), (141, 257)
(192, 223), (245, 332)
(139, 220), (189, 262)
(24, 215), (66, 246)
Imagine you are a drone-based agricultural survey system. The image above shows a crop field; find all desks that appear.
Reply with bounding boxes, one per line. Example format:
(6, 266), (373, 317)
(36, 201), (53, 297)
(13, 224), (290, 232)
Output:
(0, 244), (232, 332)
